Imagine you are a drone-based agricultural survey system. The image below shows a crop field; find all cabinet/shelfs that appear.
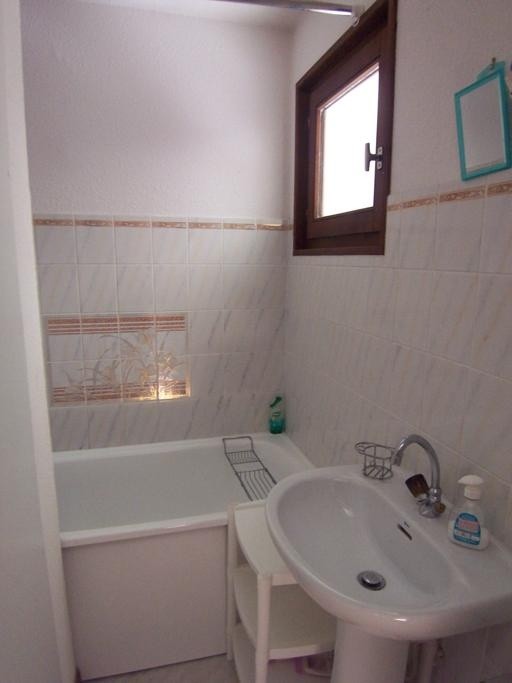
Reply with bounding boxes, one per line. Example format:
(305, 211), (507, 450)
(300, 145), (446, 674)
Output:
(223, 504), (336, 683)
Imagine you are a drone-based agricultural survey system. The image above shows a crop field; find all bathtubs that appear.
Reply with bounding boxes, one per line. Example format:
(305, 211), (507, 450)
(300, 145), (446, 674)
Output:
(49, 431), (314, 683)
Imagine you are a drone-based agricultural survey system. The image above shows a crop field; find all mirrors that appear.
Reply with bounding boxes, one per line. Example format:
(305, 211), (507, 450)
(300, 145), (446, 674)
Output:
(451, 56), (512, 182)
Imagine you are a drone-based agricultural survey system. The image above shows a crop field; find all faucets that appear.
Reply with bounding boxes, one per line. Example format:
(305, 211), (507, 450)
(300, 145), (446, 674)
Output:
(389, 434), (447, 519)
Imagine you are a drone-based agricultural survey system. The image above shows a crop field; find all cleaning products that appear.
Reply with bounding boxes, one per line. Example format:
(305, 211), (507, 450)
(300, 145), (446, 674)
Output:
(269, 395), (285, 434)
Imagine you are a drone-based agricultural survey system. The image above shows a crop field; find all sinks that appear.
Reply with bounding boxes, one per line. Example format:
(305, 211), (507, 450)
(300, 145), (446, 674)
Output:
(267, 455), (511, 639)
